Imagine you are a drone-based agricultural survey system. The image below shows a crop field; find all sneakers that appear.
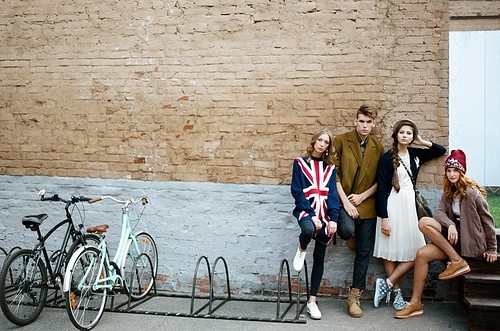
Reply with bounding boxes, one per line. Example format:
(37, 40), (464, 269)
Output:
(293, 246), (306, 272)
(306, 302), (322, 319)
(391, 288), (406, 310)
(374, 278), (389, 308)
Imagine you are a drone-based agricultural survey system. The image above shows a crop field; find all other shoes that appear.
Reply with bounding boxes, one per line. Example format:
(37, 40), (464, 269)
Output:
(394, 303), (424, 319)
(438, 258), (471, 280)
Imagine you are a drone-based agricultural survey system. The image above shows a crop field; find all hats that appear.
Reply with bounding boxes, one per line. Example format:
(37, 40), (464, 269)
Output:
(392, 119), (419, 137)
(444, 149), (466, 175)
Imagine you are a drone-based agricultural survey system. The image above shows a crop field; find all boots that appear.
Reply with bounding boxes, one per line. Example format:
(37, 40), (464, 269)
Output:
(347, 235), (356, 251)
(347, 286), (363, 318)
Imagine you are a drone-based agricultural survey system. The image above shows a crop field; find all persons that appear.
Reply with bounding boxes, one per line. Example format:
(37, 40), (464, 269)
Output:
(374, 120), (447, 310)
(395, 149), (497, 318)
(328, 104), (384, 317)
(291, 127), (340, 319)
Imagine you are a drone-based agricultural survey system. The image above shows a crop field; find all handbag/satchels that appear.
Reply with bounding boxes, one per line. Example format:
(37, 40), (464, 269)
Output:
(411, 189), (433, 221)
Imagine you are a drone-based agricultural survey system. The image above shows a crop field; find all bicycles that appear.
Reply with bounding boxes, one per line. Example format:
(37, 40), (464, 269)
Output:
(0, 188), (95, 327)
(63, 194), (159, 331)
